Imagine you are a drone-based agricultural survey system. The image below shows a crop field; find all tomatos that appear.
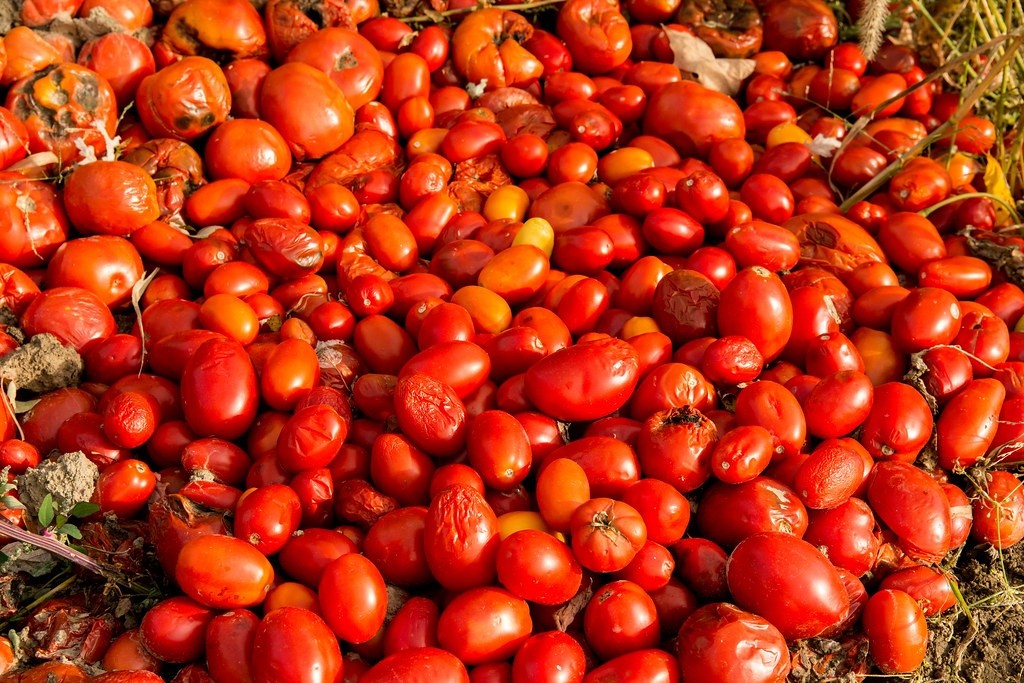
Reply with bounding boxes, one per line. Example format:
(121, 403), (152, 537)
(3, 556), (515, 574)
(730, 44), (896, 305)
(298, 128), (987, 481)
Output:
(0, 0), (1024, 683)
